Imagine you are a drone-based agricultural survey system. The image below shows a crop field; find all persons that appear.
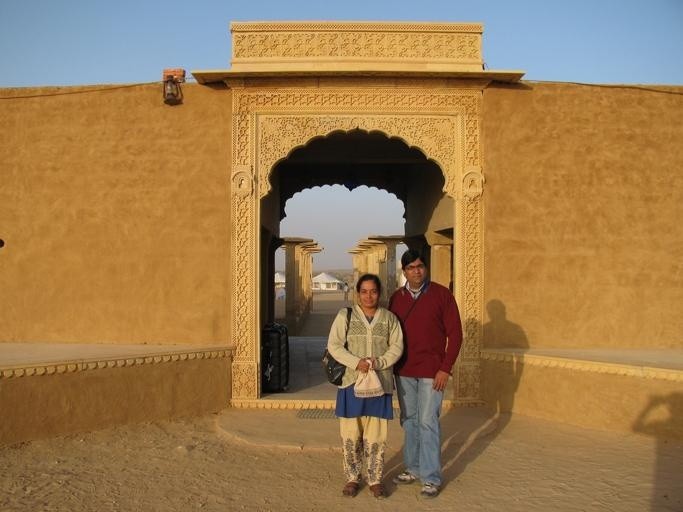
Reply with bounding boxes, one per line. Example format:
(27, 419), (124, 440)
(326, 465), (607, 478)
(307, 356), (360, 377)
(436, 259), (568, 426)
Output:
(327, 249), (462, 500)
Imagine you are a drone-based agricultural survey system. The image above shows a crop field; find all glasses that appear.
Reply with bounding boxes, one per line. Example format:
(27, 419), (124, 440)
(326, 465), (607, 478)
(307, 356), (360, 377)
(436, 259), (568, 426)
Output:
(403, 264), (425, 270)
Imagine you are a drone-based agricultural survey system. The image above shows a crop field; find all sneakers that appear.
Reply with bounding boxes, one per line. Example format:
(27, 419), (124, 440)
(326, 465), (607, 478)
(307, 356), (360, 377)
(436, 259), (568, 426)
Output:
(392, 469), (418, 484)
(419, 482), (441, 498)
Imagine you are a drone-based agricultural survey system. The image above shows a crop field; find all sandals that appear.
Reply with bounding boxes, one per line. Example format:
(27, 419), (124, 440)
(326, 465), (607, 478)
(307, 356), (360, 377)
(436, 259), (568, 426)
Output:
(342, 480), (358, 497)
(369, 482), (387, 501)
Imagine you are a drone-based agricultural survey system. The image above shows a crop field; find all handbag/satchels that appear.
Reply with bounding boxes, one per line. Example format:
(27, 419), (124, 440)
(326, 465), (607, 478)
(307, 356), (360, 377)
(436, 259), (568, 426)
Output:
(322, 346), (345, 385)
